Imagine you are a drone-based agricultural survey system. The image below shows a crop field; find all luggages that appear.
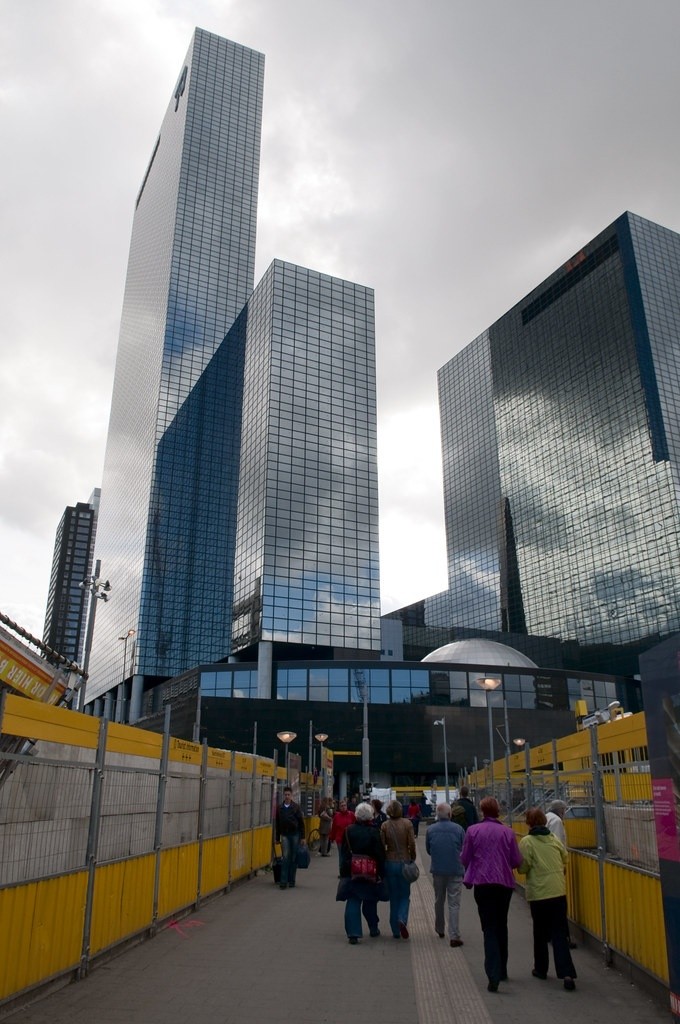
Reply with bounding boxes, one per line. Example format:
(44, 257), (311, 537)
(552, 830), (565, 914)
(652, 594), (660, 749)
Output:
(272, 842), (284, 883)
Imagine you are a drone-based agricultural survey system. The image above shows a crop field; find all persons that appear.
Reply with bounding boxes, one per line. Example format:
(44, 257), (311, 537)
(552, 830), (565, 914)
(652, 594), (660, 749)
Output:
(380, 799), (416, 940)
(338, 801), (386, 943)
(318, 793), (387, 858)
(408, 797), (421, 837)
(460, 797), (522, 991)
(516, 809), (578, 991)
(275, 786), (306, 889)
(456, 786), (480, 828)
(425, 801), (466, 948)
(329, 799), (356, 879)
(544, 799), (578, 950)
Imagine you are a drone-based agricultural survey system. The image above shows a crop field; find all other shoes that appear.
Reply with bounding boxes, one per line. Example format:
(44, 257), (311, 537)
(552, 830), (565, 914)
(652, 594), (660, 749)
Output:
(322, 854), (331, 857)
(289, 883), (295, 887)
(396, 919), (409, 939)
(280, 883), (287, 888)
(349, 938), (358, 944)
(371, 930), (380, 937)
(435, 928), (444, 938)
(450, 940), (463, 947)
(488, 978), (499, 992)
(532, 969), (547, 980)
(394, 935), (400, 938)
(564, 976), (576, 991)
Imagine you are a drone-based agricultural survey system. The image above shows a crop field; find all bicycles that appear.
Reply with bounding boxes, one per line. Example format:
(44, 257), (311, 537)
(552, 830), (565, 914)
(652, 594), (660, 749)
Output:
(308, 827), (322, 851)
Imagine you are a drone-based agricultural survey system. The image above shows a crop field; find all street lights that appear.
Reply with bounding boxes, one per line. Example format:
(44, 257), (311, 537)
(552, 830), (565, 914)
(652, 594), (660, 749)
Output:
(79, 560), (112, 712)
(434, 715), (451, 803)
(512, 735), (524, 811)
(475, 677), (501, 798)
(118, 629), (138, 723)
(278, 731), (297, 788)
(316, 733), (329, 799)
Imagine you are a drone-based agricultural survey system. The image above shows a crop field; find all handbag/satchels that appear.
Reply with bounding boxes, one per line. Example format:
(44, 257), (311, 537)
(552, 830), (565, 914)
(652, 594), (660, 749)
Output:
(297, 841), (310, 869)
(320, 807), (331, 822)
(351, 855), (377, 883)
(402, 863), (420, 883)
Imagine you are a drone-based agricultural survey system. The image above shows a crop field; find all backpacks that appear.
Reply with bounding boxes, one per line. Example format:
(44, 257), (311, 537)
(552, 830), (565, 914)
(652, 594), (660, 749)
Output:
(451, 801), (467, 829)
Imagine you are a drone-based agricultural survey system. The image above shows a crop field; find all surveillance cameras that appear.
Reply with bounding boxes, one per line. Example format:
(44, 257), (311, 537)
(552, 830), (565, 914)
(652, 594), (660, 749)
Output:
(434, 722), (439, 726)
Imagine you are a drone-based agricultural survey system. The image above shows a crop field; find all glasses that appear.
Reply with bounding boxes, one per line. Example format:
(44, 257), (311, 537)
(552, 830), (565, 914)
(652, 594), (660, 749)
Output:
(341, 805), (345, 807)
